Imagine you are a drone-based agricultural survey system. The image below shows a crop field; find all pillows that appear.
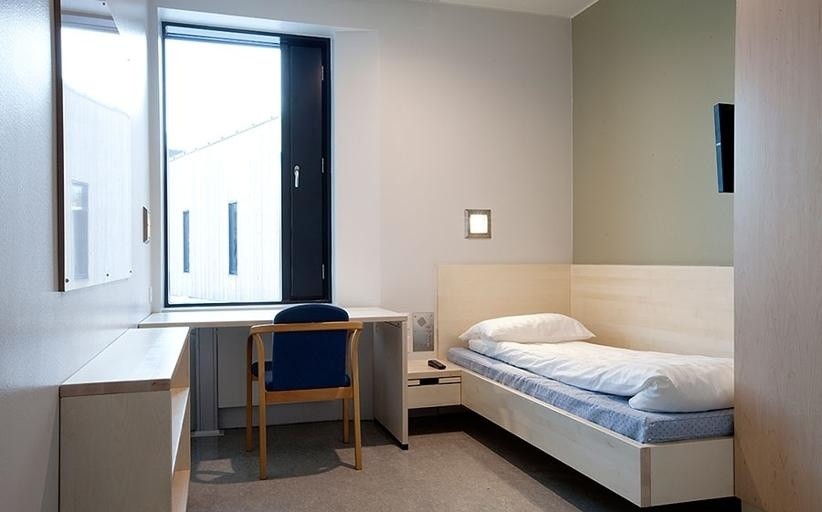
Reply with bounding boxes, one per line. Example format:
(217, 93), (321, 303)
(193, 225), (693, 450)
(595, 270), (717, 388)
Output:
(459, 313), (596, 344)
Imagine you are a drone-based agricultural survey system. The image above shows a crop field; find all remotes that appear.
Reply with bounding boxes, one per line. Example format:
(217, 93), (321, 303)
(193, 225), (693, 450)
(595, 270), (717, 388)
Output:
(428, 359), (446, 369)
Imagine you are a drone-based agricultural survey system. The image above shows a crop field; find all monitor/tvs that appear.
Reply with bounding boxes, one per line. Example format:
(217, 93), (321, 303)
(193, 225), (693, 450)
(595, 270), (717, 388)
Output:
(713, 103), (735, 193)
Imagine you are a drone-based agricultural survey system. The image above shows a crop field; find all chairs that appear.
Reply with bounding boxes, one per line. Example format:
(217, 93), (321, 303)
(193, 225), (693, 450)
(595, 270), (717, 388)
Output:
(239, 303), (362, 479)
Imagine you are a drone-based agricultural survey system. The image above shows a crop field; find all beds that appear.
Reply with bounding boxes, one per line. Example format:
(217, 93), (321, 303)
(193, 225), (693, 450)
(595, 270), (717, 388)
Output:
(434, 262), (737, 509)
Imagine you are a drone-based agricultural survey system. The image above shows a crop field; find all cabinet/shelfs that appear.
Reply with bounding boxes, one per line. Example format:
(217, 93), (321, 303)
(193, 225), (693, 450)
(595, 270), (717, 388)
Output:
(57, 326), (191, 510)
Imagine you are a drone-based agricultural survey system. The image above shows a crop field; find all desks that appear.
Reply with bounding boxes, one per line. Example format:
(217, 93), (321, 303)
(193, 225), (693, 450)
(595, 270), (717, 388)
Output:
(139, 303), (412, 451)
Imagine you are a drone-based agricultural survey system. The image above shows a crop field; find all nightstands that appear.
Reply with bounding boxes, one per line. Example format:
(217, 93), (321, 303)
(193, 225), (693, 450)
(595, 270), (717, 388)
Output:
(407, 356), (460, 409)
